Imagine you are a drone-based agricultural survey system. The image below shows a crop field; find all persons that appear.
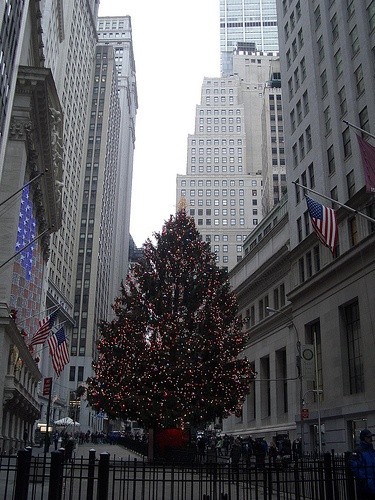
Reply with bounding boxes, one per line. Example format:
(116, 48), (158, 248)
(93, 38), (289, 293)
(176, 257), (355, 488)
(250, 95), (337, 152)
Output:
(350, 430), (375, 500)
(198, 432), (301, 470)
(23, 429), (28, 446)
(55, 429), (141, 445)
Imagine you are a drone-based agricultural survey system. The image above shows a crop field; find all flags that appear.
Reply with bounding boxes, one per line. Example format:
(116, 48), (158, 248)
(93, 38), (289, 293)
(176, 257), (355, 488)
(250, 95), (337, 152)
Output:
(32, 308), (60, 345)
(356, 134), (375, 192)
(48, 326), (70, 377)
(305, 195), (338, 255)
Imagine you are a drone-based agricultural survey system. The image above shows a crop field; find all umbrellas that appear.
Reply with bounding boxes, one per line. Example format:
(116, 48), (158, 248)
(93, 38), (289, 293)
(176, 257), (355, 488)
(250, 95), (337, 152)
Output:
(55, 417), (80, 432)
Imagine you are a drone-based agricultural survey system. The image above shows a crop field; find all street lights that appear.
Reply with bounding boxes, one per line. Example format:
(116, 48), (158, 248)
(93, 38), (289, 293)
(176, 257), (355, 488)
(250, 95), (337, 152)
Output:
(301, 389), (323, 457)
(266, 306), (305, 456)
